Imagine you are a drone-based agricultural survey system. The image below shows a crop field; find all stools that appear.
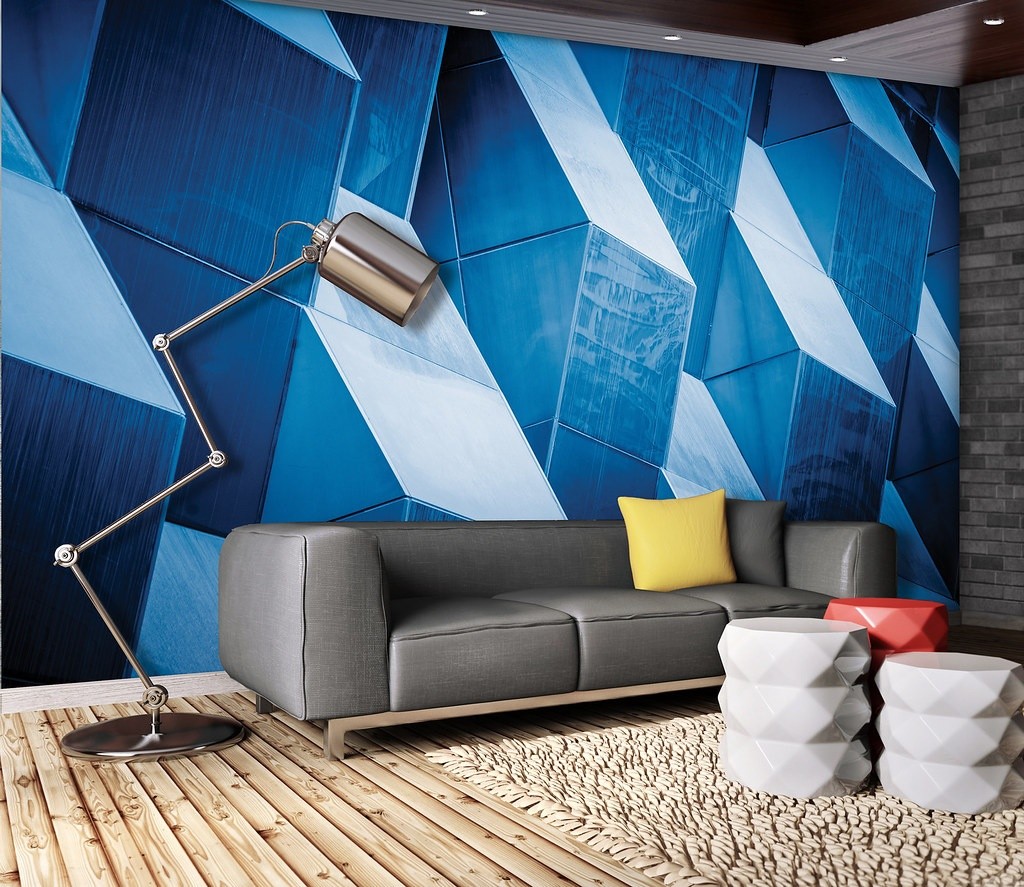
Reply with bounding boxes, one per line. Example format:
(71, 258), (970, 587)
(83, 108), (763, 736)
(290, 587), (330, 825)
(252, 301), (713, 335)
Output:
(874, 651), (1024, 814)
(822, 596), (950, 715)
(717, 616), (876, 796)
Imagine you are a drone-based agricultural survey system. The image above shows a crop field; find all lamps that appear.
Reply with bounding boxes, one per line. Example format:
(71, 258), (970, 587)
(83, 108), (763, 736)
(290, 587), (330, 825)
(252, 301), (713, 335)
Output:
(50, 211), (441, 762)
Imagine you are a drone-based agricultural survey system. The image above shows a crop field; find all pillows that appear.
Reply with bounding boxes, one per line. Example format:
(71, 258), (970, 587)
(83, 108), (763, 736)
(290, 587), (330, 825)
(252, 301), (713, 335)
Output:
(727, 498), (787, 587)
(617, 488), (737, 593)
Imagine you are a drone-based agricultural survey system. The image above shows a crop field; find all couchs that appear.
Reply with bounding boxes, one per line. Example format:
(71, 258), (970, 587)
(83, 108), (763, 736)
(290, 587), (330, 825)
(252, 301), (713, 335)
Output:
(217, 501), (897, 759)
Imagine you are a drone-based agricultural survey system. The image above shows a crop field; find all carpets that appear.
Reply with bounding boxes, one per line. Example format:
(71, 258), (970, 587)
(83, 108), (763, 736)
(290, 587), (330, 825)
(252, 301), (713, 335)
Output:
(428, 712), (1024, 886)
